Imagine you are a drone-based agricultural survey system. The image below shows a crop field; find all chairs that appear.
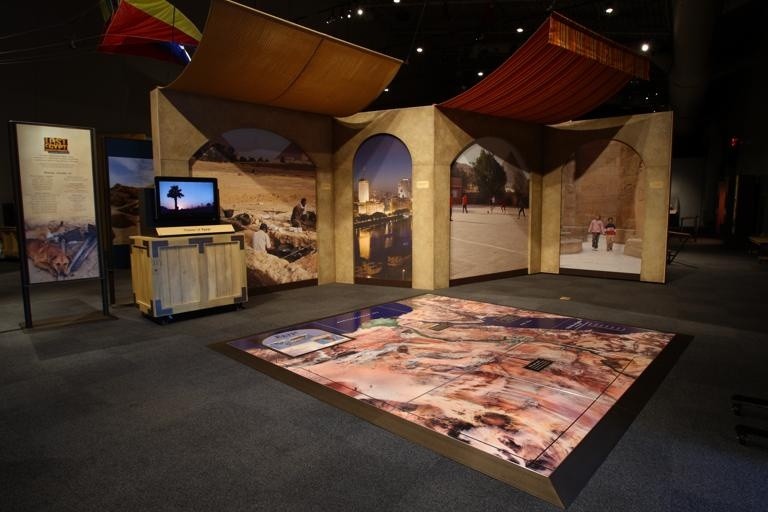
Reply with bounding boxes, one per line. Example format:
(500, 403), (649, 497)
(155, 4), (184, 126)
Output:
(681, 217), (697, 243)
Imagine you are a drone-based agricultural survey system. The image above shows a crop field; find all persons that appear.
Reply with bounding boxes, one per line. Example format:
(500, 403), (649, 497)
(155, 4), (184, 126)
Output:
(252, 223), (272, 254)
(450, 191), (526, 221)
(604, 217), (616, 251)
(588, 214), (605, 251)
(291, 198), (306, 227)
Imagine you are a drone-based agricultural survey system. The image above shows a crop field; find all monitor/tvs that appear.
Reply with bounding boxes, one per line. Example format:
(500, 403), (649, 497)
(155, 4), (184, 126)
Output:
(153, 176), (220, 226)
